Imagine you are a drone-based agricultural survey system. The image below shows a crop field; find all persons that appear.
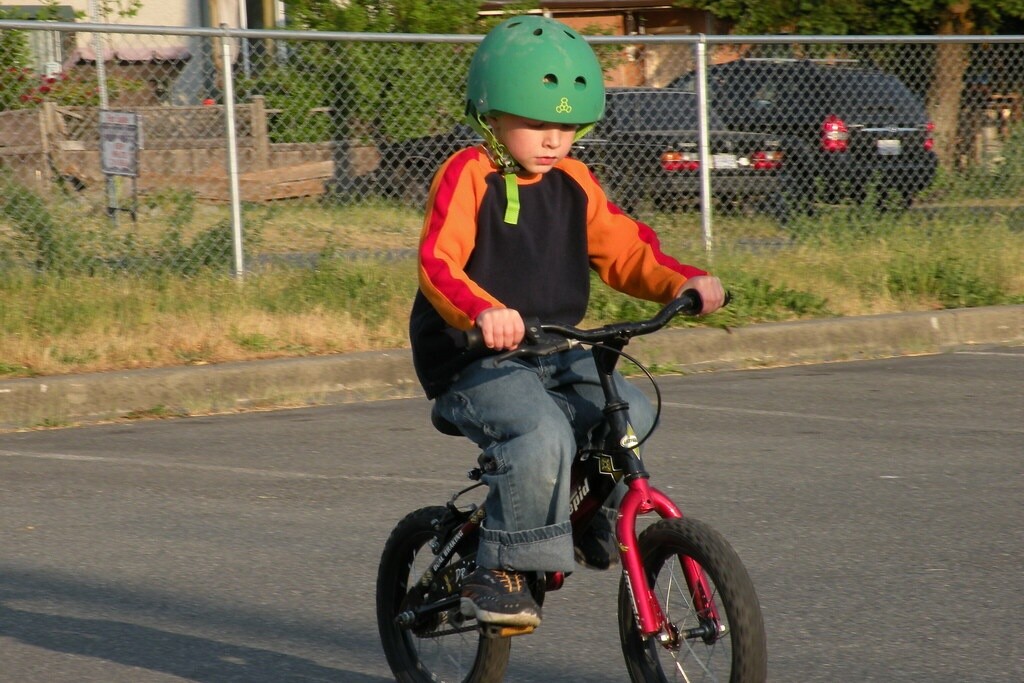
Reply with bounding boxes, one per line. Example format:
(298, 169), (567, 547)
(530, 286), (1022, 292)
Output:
(410, 16), (726, 627)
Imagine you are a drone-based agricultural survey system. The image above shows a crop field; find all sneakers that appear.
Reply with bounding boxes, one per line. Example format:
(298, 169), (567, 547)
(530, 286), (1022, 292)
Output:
(571, 519), (620, 571)
(460, 566), (541, 627)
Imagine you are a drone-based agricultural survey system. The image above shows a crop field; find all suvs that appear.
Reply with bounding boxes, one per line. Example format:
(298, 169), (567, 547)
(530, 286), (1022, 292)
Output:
(665, 58), (939, 212)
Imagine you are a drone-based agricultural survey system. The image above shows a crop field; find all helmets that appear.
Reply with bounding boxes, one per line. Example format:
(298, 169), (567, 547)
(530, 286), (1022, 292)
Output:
(464, 15), (605, 123)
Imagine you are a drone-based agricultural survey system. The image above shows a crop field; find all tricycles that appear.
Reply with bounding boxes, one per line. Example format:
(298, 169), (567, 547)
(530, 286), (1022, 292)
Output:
(375, 287), (770, 682)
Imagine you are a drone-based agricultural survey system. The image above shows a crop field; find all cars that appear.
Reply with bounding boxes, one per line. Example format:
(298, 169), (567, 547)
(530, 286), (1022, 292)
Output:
(370, 82), (813, 218)
